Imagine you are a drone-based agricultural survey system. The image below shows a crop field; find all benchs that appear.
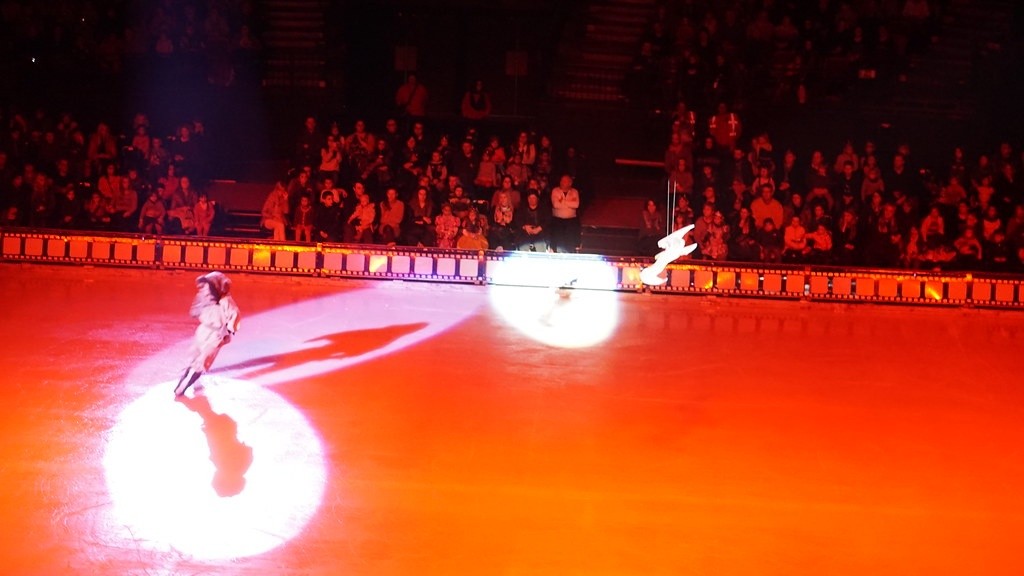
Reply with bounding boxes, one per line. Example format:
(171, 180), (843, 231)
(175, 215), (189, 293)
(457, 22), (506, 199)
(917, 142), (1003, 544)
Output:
(2, 1), (1022, 280)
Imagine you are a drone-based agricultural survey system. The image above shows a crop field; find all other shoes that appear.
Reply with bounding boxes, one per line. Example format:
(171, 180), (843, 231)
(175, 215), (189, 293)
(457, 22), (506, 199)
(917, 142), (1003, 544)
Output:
(175, 368), (205, 396)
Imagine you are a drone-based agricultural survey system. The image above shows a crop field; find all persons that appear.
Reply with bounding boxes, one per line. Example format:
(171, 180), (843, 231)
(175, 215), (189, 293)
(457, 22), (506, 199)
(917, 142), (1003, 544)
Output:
(185, 271), (240, 372)
(0, 0), (1024, 269)
